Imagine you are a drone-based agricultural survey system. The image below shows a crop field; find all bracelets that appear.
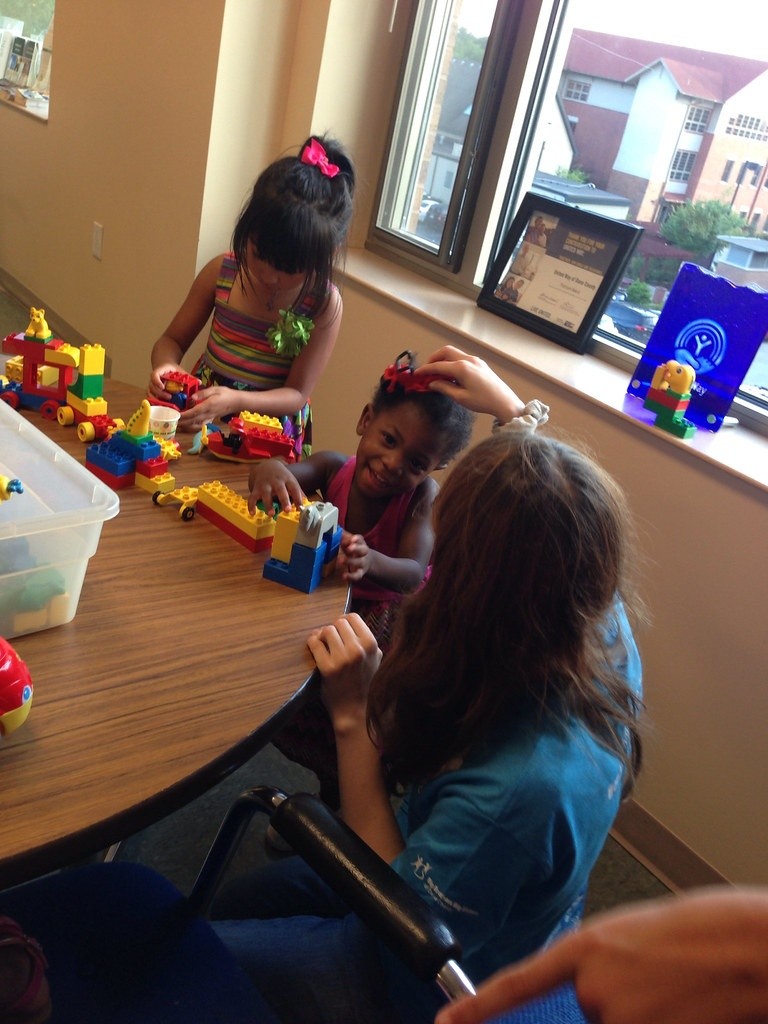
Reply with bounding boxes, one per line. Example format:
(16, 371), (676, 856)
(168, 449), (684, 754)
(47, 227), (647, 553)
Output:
(491, 400), (550, 435)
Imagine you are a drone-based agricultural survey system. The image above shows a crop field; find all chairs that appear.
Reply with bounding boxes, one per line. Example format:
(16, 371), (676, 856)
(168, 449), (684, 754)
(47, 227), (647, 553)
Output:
(0, 786), (477, 1024)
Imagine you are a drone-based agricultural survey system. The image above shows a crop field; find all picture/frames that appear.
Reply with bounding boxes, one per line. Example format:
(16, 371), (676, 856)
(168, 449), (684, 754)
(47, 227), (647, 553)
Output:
(476, 191), (644, 357)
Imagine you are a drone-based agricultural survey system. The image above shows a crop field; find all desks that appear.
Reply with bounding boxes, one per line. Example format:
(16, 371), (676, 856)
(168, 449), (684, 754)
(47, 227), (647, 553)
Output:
(0, 377), (352, 892)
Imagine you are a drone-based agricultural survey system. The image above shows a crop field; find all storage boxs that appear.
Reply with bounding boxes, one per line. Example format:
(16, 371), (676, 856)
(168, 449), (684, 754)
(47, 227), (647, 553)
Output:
(0, 397), (121, 640)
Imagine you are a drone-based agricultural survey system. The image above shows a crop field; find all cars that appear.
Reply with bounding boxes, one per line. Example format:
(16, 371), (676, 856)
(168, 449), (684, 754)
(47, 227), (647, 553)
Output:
(605, 300), (662, 346)
(419, 199), (449, 232)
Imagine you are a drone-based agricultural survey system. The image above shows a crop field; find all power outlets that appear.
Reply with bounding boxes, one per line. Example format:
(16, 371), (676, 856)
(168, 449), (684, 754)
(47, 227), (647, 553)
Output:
(91, 221), (104, 260)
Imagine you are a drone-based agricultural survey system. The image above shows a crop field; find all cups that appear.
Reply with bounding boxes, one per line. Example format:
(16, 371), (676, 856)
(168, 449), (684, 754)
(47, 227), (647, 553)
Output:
(149, 406), (181, 441)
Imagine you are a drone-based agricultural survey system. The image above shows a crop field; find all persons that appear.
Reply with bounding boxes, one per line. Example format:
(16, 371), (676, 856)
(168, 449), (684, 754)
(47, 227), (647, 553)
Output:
(525, 217), (546, 248)
(248, 350), (476, 851)
(150, 135), (355, 463)
(512, 244), (528, 276)
(496, 277), (525, 302)
(203, 345), (643, 1024)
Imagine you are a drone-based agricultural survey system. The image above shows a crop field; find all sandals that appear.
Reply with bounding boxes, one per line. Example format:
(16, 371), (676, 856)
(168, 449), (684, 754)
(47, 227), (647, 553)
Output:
(0, 915), (52, 1024)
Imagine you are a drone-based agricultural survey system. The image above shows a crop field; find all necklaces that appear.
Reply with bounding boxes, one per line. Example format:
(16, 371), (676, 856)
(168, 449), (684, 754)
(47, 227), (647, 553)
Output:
(266, 292), (276, 310)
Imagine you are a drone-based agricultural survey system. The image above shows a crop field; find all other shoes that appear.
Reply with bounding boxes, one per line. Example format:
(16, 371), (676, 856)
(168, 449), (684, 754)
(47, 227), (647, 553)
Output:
(263, 794), (344, 852)
